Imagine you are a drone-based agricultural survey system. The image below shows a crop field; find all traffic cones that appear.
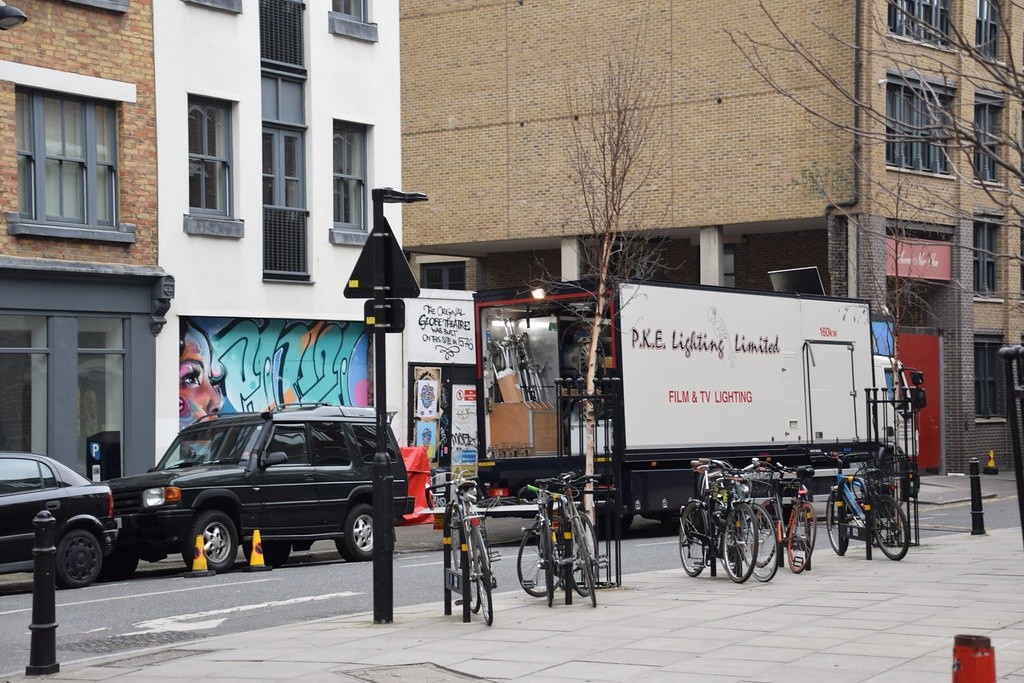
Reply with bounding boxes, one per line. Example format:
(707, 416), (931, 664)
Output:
(983, 450), (998, 475)
(184, 534), (215, 578)
(242, 529), (273, 572)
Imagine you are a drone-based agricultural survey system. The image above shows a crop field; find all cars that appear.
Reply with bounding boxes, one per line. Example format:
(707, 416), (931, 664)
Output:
(0, 451), (119, 591)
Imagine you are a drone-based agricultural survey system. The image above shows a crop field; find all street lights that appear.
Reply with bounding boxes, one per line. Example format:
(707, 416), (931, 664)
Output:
(372, 187), (429, 624)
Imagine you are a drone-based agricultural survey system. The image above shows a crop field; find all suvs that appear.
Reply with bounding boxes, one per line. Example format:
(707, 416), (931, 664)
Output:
(99, 402), (415, 580)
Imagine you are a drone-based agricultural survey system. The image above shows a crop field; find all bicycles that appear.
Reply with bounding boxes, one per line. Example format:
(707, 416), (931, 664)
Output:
(517, 471), (608, 609)
(680, 447), (912, 583)
(424, 470), (500, 627)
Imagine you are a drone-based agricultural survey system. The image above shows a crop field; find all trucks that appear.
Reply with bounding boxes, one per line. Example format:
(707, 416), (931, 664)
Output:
(471, 274), (927, 537)
